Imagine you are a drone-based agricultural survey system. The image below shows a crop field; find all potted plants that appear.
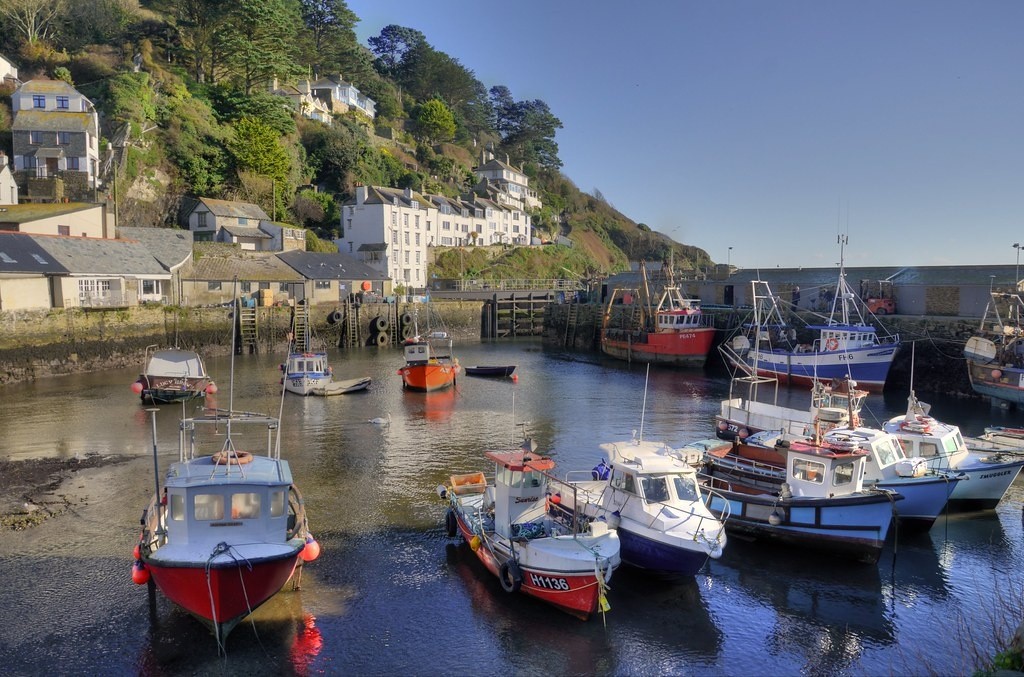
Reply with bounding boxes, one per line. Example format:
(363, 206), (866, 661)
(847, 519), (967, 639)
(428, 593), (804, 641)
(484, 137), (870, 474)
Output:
(63, 196), (70, 203)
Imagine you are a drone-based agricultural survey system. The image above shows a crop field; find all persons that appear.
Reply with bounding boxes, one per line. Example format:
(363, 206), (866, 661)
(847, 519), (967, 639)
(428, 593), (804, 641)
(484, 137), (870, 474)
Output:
(298, 298), (307, 305)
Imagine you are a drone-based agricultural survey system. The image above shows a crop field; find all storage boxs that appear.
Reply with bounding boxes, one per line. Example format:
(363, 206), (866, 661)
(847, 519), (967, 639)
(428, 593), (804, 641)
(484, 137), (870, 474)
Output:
(450, 472), (487, 494)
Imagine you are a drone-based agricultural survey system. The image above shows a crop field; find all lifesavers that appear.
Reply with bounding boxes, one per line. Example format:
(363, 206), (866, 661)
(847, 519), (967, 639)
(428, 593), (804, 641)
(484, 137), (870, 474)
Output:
(374, 332), (388, 346)
(333, 311), (343, 322)
(901, 415), (935, 433)
(400, 313), (412, 326)
(211, 449), (252, 465)
(498, 559), (522, 593)
(826, 338), (838, 350)
(445, 510), (457, 539)
(376, 317), (389, 331)
(400, 326), (412, 340)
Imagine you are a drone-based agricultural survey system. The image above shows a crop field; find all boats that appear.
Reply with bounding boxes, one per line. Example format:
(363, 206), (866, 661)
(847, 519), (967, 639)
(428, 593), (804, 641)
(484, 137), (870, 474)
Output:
(130, 302), (219, 404)
(882, 339), (1023, 513)
(823, 372), (970, 537)
(713, 302), (870, 442)
(129, 274), (320, 657)
(591, 363), (732, 585)
(396, 289), (461, 391)
(682, 430), (906, 562)
(718, 195), (902, 395)
(435, 391), (623, 621)
(313, 376), (372, 396)
(961, 242), (1024, 403)
(464, 365), (516, 377)
(601, 249), (718, 369)
(276, 295), (334, 395)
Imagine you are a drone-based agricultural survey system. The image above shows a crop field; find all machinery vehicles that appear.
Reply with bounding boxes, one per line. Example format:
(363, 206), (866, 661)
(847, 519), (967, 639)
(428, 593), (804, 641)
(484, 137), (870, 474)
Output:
(858, 278), (897, 315)
